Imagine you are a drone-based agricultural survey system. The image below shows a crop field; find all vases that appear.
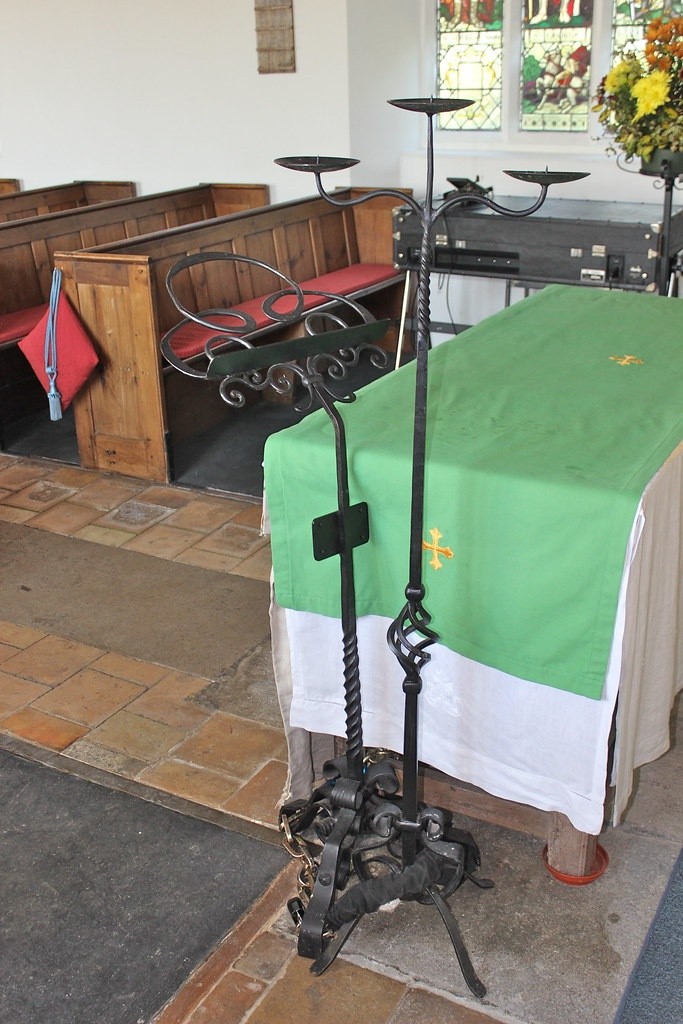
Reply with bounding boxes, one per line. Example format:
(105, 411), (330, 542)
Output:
(638, 147), (683, 173)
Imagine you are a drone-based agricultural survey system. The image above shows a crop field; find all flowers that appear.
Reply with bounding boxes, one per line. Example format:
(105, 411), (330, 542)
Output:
(592, 17), (683, 160)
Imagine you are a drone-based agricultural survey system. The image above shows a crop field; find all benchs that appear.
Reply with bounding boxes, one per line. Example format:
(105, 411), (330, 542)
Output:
(0, 174), (413, 481)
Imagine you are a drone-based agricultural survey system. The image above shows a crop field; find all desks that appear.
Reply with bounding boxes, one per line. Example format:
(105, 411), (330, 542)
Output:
(260, 286), (683, 882)
